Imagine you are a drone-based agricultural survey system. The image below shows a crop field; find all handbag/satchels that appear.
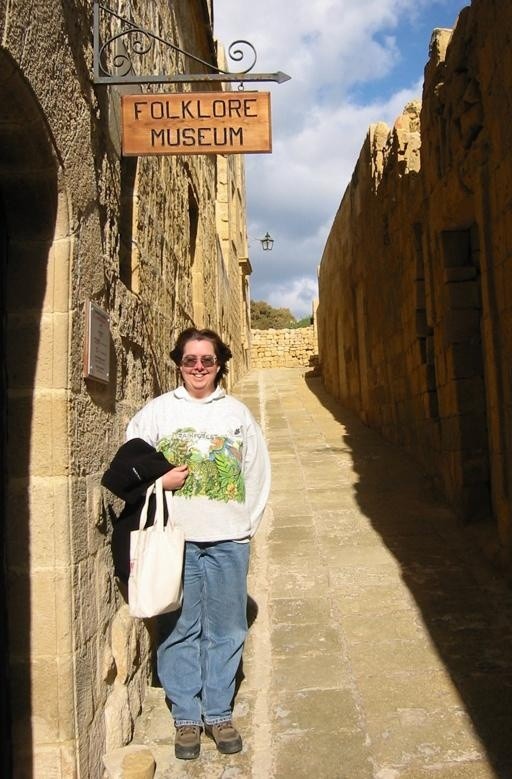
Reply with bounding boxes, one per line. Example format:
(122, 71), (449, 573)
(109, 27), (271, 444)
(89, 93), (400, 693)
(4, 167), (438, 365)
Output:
(128, 525), (185, 620)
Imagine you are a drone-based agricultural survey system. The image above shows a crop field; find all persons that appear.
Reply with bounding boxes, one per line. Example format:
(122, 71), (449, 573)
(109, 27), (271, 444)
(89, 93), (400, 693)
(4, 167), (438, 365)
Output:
(124, 329), (269, 759)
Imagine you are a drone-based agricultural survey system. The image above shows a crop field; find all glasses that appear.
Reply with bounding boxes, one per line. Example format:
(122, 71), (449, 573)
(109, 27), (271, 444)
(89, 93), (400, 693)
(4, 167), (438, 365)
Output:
(179, 355), (217, 367)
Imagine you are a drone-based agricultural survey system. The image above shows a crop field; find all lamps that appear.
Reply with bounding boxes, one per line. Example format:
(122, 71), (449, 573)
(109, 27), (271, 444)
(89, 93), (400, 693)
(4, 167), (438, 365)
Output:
(247, 232), (275, 252)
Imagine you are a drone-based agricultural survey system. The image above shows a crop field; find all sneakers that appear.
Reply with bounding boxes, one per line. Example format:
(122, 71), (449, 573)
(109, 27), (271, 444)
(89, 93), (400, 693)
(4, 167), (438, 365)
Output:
(175, 725), (201, 760)
(204, 720), (242, 754)
